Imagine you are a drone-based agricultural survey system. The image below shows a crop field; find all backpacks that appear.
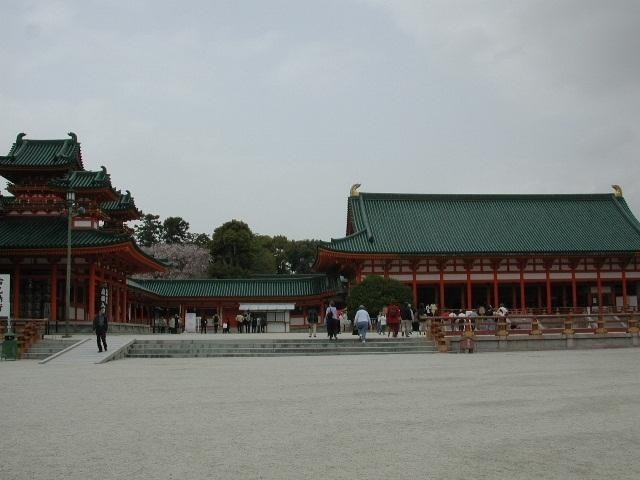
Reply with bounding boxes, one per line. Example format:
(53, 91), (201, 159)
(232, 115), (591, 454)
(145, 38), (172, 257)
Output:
(214, 315), (219, 323)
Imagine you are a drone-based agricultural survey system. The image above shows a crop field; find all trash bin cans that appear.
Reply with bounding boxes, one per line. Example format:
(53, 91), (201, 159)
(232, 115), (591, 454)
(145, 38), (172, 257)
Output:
(2, 334), (17, 361)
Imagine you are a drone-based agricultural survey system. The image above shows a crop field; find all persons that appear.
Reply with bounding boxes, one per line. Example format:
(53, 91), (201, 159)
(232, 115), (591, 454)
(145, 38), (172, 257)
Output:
(353, 304), (372, 344)
(307, 305), (319, 337)
(154, 310), (267, 334)
(92, 306), (109, 353)
(324, 300), (341, 341)
(376, 301), (510, 340)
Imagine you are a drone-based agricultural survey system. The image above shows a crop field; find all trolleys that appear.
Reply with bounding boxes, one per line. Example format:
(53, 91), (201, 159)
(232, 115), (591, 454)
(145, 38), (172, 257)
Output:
(410, 320), (422, 335)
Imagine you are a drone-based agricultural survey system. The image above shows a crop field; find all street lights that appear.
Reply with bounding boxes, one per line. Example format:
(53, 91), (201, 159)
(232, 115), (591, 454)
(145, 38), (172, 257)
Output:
(63, 187), (76, 337)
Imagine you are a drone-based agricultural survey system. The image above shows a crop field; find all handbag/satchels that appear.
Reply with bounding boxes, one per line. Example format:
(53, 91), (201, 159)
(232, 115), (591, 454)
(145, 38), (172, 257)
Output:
(419, 315), (427, 321)
(327, 311), (333, 318)
(352, 329), (359, 335)
